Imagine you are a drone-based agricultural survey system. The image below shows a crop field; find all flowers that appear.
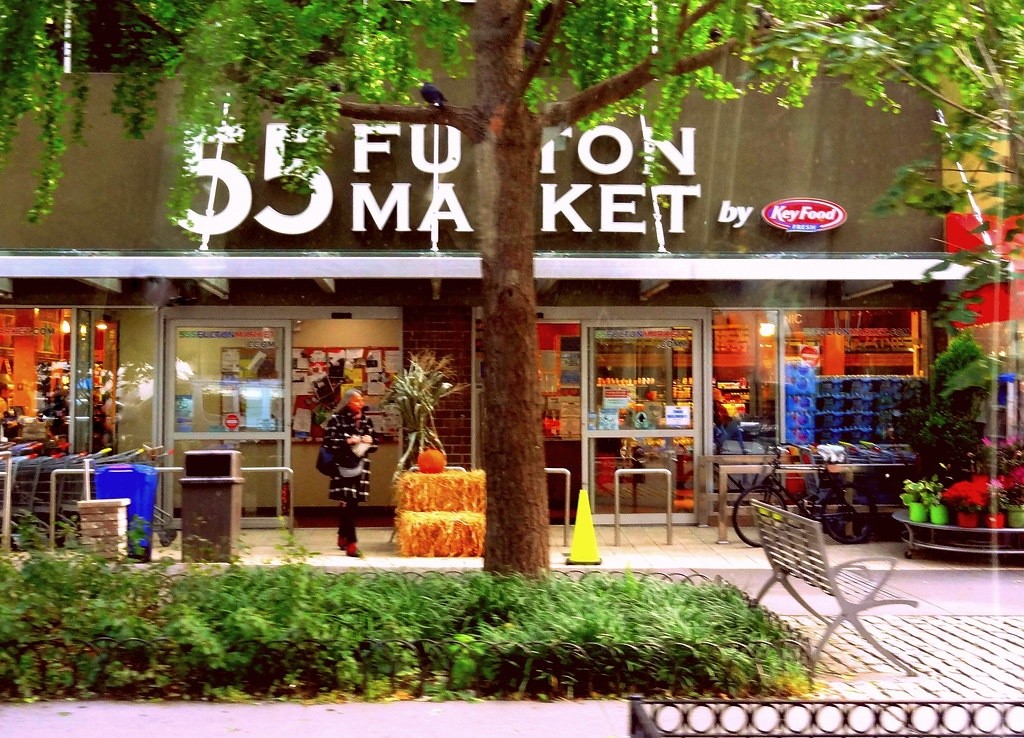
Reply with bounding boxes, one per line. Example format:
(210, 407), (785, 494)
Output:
(941, 476), (989, 510)
(986, 479), (1007, 511)
(1001, 466), (1024, 505)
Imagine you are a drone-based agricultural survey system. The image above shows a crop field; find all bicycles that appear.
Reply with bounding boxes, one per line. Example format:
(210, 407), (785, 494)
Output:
(732, 444), (877, 549)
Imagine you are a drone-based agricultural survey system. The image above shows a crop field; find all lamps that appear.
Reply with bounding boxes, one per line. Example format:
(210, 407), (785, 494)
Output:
(419, 84), (448, 110)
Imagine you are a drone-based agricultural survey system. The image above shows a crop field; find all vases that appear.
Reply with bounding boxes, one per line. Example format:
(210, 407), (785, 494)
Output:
(1006, 505), (1024, 528)
(959, 512), (978, 527)
(986, 513), (1005, 529)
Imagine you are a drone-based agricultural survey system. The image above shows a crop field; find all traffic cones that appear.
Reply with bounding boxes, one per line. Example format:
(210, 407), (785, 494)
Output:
(566, 489), (603, 566)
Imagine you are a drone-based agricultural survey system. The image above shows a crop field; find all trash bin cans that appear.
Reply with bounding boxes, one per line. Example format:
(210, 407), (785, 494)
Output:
(93, 464), (162, 560)
(178, 449), (246, 564)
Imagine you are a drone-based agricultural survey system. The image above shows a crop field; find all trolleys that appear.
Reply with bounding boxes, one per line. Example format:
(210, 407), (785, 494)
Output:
(0, 438), (178, 550)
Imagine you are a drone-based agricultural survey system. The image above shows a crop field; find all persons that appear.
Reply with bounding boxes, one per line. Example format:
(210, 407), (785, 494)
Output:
(761, 399), (775, 438)
(323, 389), (378, 557)
(713, 388), (729, 441)
(0, 382), (10, 419)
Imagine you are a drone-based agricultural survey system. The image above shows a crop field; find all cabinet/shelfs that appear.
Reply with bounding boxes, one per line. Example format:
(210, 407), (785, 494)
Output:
(892, 509), (1024, 560)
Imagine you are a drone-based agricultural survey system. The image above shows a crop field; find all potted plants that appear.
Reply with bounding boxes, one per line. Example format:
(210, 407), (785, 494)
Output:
(925, 475), (948, 525)
(901, 480), (927, 521)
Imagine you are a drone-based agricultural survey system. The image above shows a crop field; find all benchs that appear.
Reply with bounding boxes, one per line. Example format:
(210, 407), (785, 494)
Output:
(748, 500), (919, 677)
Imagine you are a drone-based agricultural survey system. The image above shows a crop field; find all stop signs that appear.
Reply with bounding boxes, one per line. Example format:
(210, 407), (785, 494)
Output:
(224, 414), (240, 429)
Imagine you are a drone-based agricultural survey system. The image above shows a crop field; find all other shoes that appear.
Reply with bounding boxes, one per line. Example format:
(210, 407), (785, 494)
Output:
(337, 533), (348, 549)
(347, 542), (362, 556)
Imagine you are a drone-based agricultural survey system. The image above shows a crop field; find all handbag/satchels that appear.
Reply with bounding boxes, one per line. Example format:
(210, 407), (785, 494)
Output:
(316, 448), (339, 477)
